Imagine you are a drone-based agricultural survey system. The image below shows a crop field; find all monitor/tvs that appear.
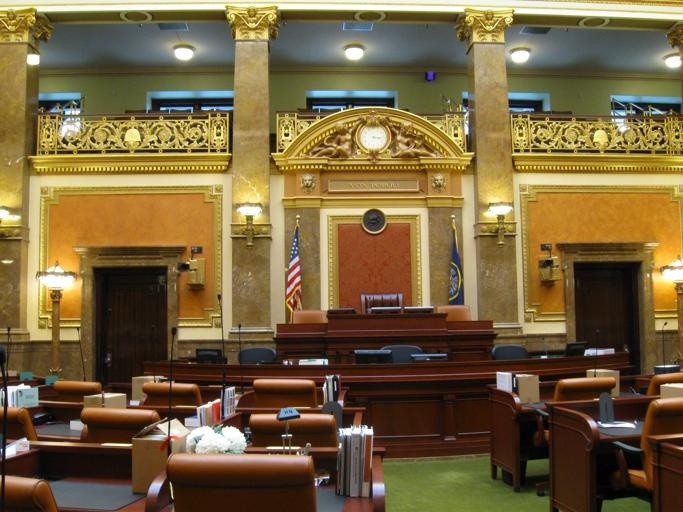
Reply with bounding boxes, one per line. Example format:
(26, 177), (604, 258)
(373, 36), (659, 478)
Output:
(564, 341), (587, 357)
(196, 348), (223, 364)
(410, 354), (447, 362)
(354, 350), (393, 364)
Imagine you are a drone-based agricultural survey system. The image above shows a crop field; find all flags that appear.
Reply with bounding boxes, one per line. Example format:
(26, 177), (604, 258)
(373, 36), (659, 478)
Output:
(285, 223), (302, 324)
(448, 222), (464, 306)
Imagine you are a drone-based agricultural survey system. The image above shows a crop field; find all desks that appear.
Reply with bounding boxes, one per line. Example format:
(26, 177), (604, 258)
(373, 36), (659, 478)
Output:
(144, 352), (631, 460)
(275, 319), (499, 365)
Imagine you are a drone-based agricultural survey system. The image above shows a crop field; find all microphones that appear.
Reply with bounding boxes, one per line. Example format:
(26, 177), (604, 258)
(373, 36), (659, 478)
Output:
(662, 321), (668, 365)
(76, 327), (87, 381)
(217, 292), (226, 419)
(237, 323), (244, 394)
(593, 327), (601, 378)
(6, 326), (12, 380)
(166, 327), (177, 503)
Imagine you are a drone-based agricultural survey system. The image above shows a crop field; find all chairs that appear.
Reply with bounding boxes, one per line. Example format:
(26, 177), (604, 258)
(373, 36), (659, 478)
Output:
(43, 380), (102, 418)
(1, 406), (38, 441)
(361, 292), (404, 314)
(245, 411), (339, 468)
(148, 451), (320, 511)
(78, 406), (161, 444)
(435, 304), (472, 322)
(292, 310), (326, 324)
(491, 344), (528, 360)
(236, 378), (317, 414)
(128, 382), (202, 416)
(548, 372), (683, 496)
(382, 345), (424, 363)
(1, 373), (48, 415)
(238, 347), (276, 364)
(0, 474), (57, 511)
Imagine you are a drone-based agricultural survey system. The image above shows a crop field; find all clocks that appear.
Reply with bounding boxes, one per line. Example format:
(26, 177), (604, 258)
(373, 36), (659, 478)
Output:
(355, 120), (392, 155)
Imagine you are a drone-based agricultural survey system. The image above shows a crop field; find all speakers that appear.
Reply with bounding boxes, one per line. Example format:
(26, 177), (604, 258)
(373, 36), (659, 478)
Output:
(187, 257), (207, 288)
(538, 255), (562, 282)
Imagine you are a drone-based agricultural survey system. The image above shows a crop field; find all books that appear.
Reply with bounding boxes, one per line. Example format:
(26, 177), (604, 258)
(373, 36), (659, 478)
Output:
(0, 383), (39, 408)
(184, 386), (236, 428)
(322, 374), (340, 405)
(335, 424), (374, 498)
(299, 358), (329, 366)
(1, 437), (29, 460)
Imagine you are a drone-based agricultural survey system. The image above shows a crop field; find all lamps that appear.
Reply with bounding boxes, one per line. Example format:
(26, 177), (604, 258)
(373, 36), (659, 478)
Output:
(27, 47), (41, 67)
(510, 47), (531, 64)
(36, 261), (78, 380)
(663, 52), (681, 68)
(488, 201), (514, 249)
(344, 43), (365, 60)
(173, 44), (197, 62)
(237, 201), (264, 250)
(660, 256), (683, 370)
